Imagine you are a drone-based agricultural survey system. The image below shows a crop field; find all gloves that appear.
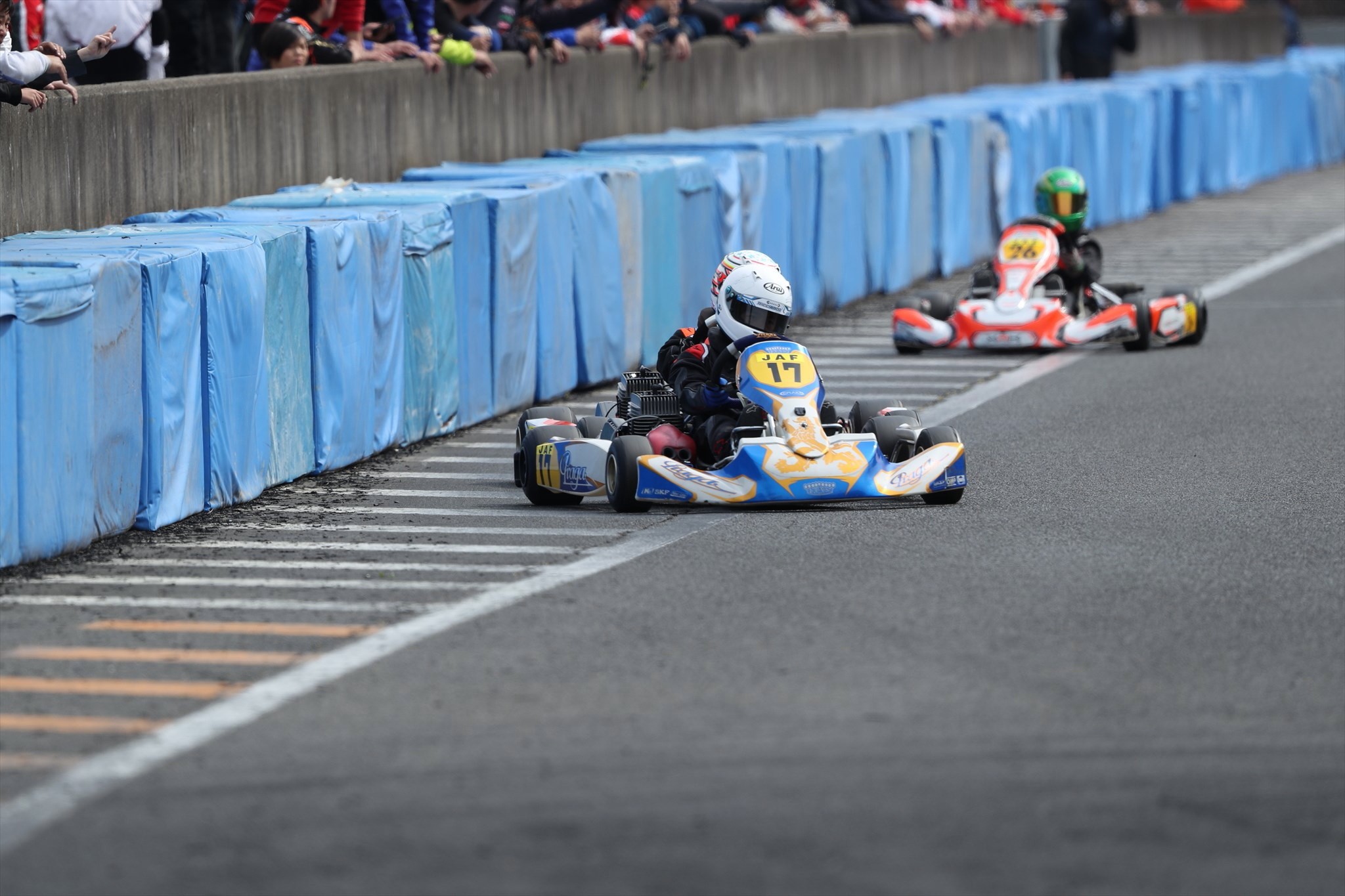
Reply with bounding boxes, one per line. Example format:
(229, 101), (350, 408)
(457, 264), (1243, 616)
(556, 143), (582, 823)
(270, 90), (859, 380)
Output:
(702, 377), (729, 409)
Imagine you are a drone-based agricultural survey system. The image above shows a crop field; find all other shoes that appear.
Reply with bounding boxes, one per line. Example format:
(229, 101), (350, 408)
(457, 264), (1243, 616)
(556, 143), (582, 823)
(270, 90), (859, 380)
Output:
(720, 403), (763, 460)
(820, 400), (837, 436)
(972, 270), (992, 297)
(1043, 274), (1066, 305)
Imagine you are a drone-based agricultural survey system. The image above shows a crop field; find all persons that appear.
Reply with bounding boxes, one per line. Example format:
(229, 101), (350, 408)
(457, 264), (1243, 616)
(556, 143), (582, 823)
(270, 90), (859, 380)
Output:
(656, 251), (837, 464)
(975, 167), (1102, 317)
(0, 0), (1302, 111)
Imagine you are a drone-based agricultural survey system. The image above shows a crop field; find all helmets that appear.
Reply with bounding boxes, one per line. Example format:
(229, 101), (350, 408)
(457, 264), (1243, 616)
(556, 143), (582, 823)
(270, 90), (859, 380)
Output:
(1034, 166), (1090, 234)
(711, 250), (782, 312)
(715, 264), (793, 350)
